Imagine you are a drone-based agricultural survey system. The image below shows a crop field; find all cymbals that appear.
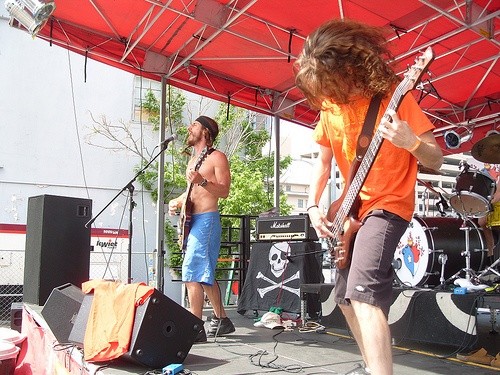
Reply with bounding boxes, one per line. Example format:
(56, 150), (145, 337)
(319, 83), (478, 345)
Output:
(418, 164), (446, 175)
(471, 133), (500, 164)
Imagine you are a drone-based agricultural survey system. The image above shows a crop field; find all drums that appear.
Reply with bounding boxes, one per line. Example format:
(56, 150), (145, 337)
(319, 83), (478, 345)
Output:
(450, 171), (496, 218)
(392, 217), (487, 288)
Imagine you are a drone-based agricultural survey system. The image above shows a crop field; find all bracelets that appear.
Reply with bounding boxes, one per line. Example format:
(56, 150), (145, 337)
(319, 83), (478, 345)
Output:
(307, 205), (318, 211)
(408, 136), (421, 152)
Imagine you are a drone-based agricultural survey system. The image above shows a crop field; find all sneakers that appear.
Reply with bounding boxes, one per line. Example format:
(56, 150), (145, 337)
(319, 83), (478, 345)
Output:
(206, 315), (235, 338)
(193, 325), (207, 344)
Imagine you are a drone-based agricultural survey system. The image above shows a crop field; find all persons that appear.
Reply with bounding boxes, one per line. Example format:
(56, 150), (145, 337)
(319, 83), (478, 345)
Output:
(168, 115), (235, 344)
(477, 129), (500, 283)
(293, 19), (443, 375)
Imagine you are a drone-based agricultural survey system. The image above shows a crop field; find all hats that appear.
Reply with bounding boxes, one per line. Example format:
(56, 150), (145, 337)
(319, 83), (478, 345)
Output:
(195, 116), (219, 138)
(261, 313), (286, 329)
(253, 312), (281, 327)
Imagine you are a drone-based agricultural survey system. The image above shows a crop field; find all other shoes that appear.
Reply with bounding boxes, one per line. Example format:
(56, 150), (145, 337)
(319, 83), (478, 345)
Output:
(344, 363), (371, 375)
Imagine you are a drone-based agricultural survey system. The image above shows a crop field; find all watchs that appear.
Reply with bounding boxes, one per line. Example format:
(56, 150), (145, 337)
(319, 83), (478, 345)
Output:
(198, 178), (208, 187)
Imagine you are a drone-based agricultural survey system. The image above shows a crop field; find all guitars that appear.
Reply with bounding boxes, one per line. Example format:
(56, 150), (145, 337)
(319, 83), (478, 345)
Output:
(177, 145), (209, 250)
(325, 46), (435, 269)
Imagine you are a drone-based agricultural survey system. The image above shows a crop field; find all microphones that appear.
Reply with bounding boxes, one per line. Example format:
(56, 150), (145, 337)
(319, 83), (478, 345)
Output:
(161, 133), (178, 145)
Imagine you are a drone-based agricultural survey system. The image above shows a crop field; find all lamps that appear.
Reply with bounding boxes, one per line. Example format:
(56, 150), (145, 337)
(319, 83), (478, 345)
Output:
(444, 126), (473, 149)
(183, 60), (197, 81)
(3, 0), (56, 40)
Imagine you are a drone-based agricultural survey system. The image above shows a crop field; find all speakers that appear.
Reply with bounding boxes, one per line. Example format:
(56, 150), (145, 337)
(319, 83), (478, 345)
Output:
(11, 194), (205, 370)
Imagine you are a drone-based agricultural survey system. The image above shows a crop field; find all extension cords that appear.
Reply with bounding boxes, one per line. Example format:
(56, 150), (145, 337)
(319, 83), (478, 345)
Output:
(162, 364), (183, 375)
